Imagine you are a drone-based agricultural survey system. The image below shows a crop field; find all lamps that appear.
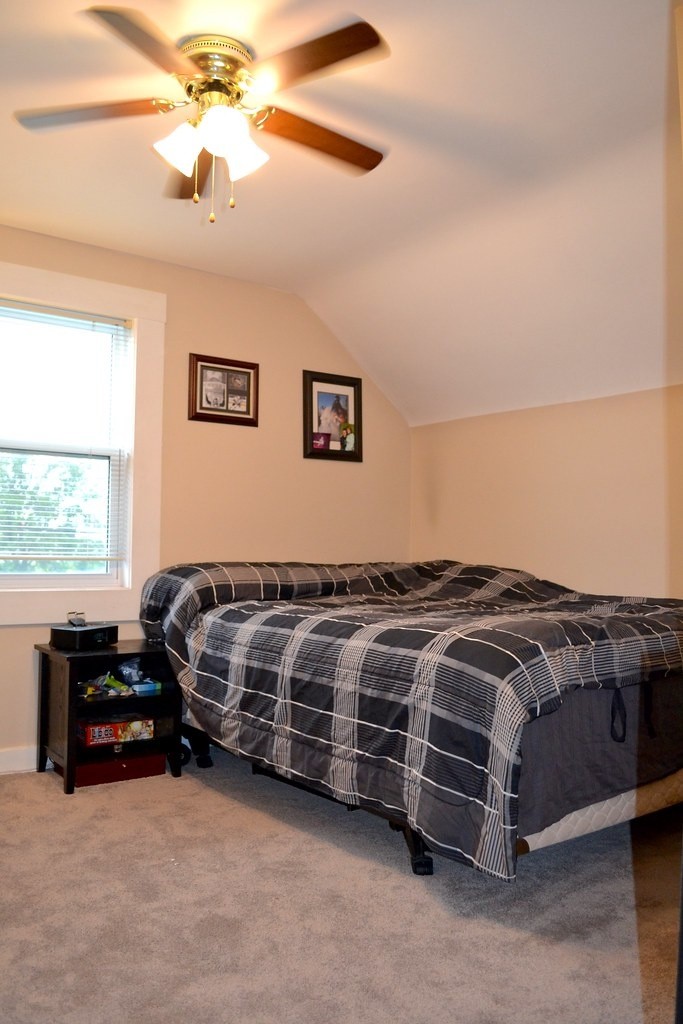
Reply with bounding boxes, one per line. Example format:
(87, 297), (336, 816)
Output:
(151, 91), (272, 222)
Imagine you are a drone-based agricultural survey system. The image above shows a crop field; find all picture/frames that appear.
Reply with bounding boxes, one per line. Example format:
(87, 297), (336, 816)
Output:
(185, 351), (260, 427)
(302, 369), (363, 462)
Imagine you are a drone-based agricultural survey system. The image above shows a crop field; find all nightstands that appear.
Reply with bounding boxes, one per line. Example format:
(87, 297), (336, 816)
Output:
(35, 642), (182, 794)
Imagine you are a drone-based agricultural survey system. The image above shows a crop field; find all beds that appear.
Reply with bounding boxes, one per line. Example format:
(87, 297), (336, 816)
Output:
(140, 559), (683, 883)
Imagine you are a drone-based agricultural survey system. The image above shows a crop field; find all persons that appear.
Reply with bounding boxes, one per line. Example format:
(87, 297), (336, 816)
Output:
(206, 395), (224, 407)
(313, 435), (324, 446)
(340, 426), (355, 451)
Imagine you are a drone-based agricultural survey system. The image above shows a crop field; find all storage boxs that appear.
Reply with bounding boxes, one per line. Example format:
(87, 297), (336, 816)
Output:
(54, 751), (166, 787)
(76, 711), (154, 748)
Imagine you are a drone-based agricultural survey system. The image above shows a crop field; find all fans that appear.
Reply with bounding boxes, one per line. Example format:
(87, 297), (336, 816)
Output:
(13, 7), (385, 200)
